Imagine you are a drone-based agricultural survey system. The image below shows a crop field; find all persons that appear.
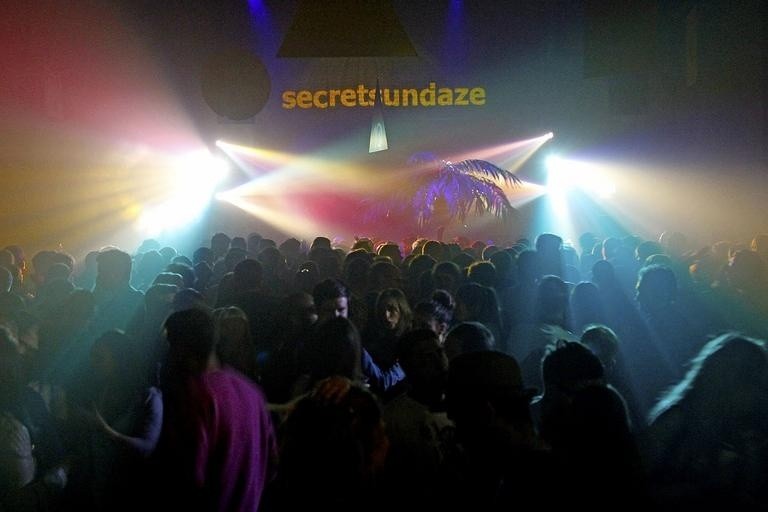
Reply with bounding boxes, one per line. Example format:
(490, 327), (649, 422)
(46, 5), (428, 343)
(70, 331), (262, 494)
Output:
(162, 309), (279, 511)
(2, 234), (766, 510)
(528, 341), (671, 509)
(643, 335), (764, 509)
